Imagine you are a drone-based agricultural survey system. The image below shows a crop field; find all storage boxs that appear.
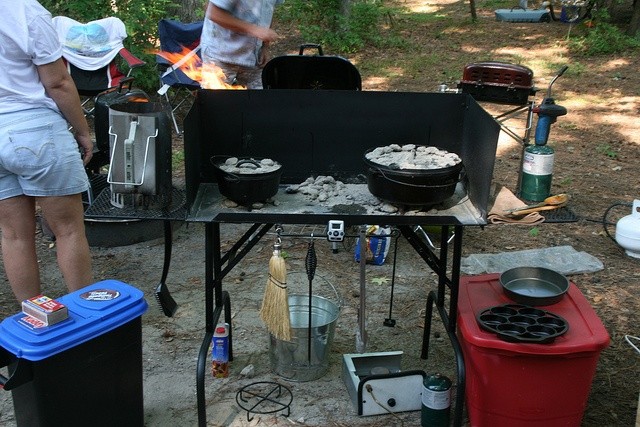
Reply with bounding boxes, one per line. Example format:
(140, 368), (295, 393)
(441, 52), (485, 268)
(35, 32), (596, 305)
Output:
(454, 273), (610, 427)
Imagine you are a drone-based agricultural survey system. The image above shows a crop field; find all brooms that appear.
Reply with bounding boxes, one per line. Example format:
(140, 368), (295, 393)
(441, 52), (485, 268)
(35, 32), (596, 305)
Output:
(260, 243), (292, 343)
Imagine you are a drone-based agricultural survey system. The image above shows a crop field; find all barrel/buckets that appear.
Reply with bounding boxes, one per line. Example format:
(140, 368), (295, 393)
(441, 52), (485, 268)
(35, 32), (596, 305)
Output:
(268, 271), (342, 382)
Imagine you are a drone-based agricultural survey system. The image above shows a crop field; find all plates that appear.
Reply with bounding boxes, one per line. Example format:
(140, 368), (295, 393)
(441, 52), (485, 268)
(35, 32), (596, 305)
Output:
(499, 267), (570, 306)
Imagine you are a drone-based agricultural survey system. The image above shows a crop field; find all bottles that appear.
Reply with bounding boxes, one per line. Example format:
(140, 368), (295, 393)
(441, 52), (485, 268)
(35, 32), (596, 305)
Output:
(211, 322), (230, 378)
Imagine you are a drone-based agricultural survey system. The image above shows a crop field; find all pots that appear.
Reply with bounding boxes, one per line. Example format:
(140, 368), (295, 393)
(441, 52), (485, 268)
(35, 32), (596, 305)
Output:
(210, 155), (283, 211)
(363, 145), (464, 206)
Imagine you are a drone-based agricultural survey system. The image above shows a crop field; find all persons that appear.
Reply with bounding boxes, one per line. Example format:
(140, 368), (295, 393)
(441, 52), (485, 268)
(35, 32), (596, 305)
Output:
(1, 0), (93, 315)
(199, 0), (280, 89)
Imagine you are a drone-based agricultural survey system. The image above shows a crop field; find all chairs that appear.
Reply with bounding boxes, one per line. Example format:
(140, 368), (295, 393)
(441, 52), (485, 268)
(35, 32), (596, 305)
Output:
(457, 61), (536, 192)
